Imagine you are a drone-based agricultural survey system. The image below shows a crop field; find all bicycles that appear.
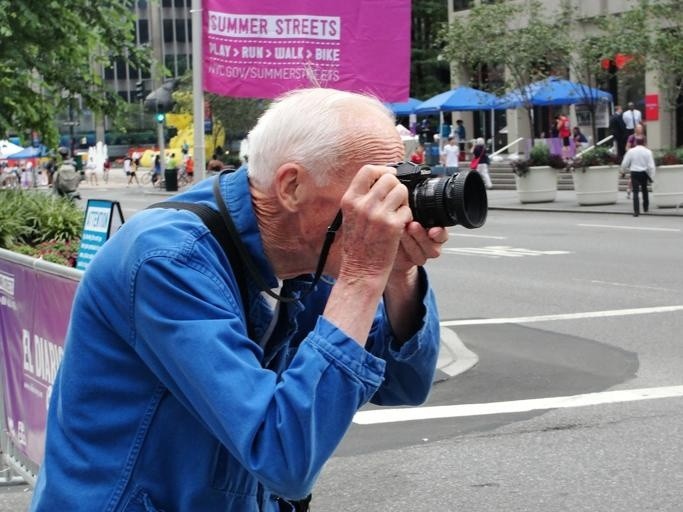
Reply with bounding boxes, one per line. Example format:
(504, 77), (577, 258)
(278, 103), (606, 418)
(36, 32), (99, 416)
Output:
(140, 171), (188, 184)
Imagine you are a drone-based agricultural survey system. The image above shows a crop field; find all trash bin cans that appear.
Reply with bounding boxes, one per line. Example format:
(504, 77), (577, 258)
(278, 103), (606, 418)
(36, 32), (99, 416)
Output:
(165, 169), (178, 191)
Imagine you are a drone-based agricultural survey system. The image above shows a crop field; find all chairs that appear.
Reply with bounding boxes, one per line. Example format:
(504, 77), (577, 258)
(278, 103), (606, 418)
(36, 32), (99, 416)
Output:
(459, 139), (477, 161)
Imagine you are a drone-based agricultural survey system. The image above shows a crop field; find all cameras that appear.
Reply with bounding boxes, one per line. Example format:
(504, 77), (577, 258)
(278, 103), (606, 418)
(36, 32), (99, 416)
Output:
(390, 160), (488, 229)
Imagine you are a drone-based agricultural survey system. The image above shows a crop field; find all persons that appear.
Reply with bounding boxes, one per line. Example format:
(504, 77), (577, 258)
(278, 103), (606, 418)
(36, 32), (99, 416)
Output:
(409, 118), (493, 190)
(31, 87), (449, 512)
(541, 100), (656, 217)
(0, 132), (250, 206)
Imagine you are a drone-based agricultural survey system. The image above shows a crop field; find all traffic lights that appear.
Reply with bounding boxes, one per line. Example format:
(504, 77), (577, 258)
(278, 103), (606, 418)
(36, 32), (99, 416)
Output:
(156, 101), (164, 123)
(136, 81), (144, 98)
